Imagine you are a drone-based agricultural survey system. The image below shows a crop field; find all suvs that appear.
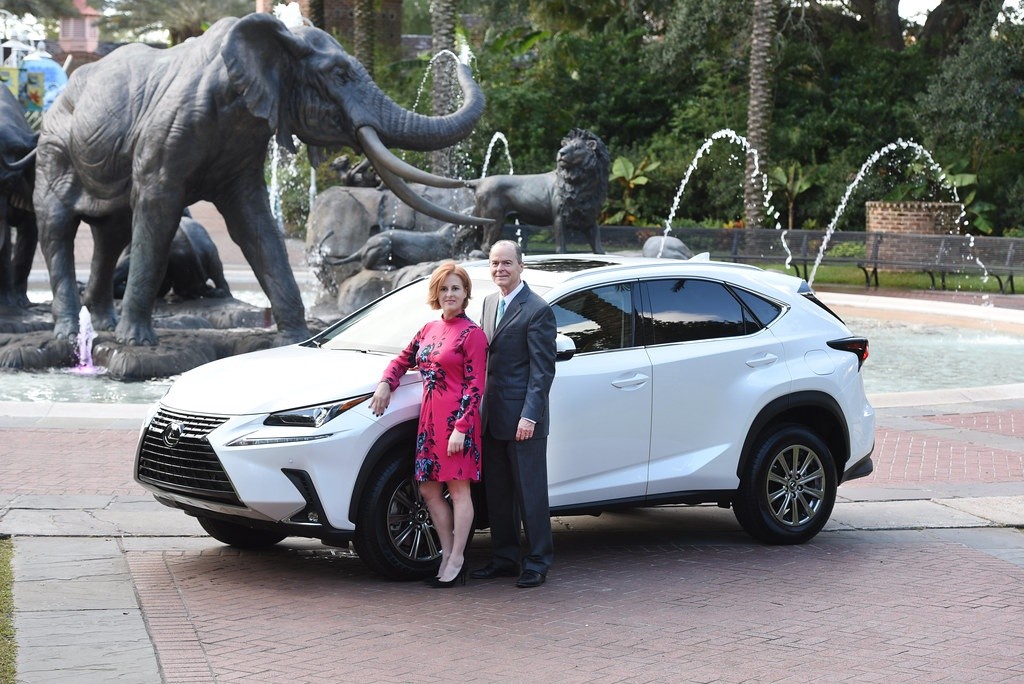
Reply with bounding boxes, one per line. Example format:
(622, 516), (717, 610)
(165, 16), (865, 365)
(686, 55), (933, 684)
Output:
(134, 252), (878, 583)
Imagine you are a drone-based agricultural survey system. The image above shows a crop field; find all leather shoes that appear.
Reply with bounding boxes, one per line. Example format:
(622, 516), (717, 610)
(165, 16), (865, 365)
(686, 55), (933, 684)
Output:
(517, 569), (546, 587)
(471, 563), (521, 579)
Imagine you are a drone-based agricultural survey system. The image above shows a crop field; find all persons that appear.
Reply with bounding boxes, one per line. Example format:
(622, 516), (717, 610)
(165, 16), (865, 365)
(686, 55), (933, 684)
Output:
(369, 259), (491, 587)
(468, 240), (560, 589)
(721, 213), (745, 251)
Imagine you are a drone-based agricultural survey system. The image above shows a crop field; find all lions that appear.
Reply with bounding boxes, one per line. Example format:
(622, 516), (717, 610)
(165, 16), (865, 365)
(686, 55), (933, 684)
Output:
(463, 124), (614, 256)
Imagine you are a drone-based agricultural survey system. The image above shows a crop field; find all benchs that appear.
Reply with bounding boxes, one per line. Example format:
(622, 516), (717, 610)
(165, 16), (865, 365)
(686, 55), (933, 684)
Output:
(597, 226), (1023, 294)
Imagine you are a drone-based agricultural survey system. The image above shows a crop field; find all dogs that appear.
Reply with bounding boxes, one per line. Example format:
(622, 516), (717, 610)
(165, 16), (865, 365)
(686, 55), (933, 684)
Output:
(318, 205), (483, 274)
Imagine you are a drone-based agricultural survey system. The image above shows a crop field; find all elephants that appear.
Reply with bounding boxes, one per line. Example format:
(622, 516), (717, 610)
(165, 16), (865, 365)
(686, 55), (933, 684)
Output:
(0, 12), (486, 352)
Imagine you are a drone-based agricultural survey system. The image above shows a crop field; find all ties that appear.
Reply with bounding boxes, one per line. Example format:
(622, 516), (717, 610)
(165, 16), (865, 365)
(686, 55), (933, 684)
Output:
(499, 300), (505, 323)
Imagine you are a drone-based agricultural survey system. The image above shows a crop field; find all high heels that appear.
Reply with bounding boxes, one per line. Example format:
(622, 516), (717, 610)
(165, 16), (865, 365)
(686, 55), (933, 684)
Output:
(422, 556), (468, 588)
